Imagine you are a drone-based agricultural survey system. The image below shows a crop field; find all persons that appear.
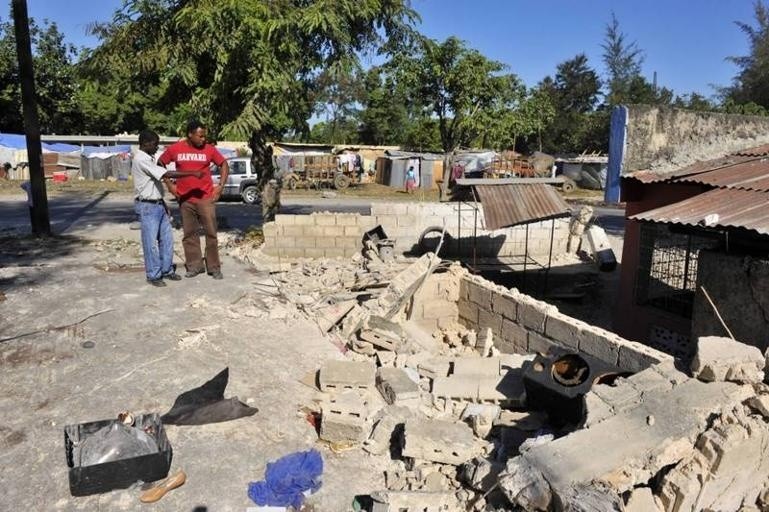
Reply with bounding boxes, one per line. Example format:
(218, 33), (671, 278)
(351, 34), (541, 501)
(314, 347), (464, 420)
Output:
(155, 121), (231, 281)
(131, 129), (206, 287)
(405, 166), (417, 195)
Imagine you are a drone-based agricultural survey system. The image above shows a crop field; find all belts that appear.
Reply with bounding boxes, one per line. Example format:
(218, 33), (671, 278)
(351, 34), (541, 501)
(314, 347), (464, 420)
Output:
(136, 198), (163, 203)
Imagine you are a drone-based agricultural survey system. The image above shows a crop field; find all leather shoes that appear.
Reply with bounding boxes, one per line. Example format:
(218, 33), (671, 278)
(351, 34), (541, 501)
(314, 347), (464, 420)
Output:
(147, 278), (166, 287)
(208, 271), (223, 279)
(163, 273), (182, 281)
(185, 269), (205, 278)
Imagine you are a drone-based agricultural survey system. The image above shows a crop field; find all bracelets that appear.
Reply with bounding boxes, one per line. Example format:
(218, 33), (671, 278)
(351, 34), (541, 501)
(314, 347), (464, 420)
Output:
(218, 183), (226, 190)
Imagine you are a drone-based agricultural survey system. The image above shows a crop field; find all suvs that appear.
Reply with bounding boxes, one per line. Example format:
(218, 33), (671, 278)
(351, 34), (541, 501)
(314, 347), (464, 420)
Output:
(208, 156), (284, 204)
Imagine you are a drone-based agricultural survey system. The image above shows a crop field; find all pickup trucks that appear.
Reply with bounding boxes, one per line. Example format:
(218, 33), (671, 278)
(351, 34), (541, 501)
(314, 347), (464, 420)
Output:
(494, 157), (551, 178)
